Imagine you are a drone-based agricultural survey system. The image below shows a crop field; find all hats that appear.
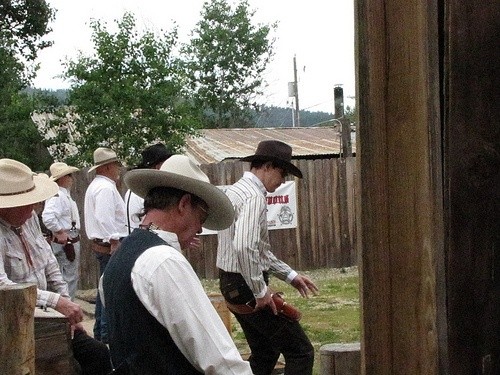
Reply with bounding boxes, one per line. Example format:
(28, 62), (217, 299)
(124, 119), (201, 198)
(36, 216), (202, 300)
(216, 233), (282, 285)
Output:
(238, 140), (305, 180)
(122, 153), (234, 232)
(136, 143), (176, 166)
(0, 158), (59, 210)
(48, 162), (80, 181)
(87, 147), (122, 172)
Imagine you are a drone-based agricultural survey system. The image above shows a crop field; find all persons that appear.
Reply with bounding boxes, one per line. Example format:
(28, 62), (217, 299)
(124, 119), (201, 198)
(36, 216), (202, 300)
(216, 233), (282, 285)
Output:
(83, 147), (126, 344)
(41, 162), (81, 302)
(124, 144), (201, 250)
(98, 154), (253, 375)
(0, 158), (113, 375)
(216, 141), (320, 375)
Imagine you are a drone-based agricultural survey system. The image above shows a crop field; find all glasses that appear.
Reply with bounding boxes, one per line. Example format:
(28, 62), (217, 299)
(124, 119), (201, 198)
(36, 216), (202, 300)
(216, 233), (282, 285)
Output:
(194, 201), (209, 224)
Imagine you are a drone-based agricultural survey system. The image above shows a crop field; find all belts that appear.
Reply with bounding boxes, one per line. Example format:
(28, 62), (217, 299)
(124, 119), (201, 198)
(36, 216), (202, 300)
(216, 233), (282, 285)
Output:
(50, 236), (81, 245)
